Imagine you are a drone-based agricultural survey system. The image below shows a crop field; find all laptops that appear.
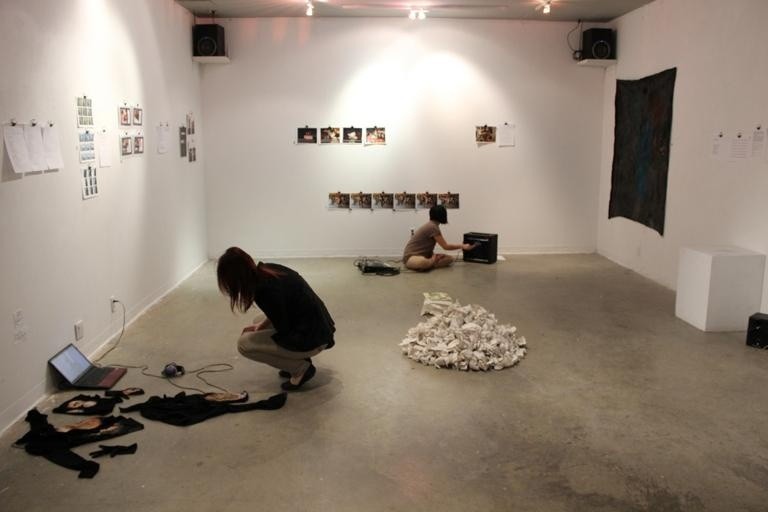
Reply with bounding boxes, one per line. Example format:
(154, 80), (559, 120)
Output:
(48, 343), (127, 390)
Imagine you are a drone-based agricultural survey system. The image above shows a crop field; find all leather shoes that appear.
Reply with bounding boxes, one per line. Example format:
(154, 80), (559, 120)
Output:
(278, 357), (316, 391)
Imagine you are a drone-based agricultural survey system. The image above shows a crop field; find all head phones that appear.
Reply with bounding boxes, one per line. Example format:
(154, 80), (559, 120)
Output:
(164, 362), (185, 377)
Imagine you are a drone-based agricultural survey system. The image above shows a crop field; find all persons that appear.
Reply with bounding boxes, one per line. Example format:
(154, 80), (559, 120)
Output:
(402, 203), (477, 272)
(216, 244), (336, 391)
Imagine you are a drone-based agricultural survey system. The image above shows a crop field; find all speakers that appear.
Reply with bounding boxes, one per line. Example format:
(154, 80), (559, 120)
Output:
(193, 24), (225, 56)
(582, 28), (616, 59)
(463, 232), (498, 264)
(746, 313), (768, 350)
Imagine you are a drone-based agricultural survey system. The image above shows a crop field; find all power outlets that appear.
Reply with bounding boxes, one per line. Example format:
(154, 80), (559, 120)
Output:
(111, 298), (115, 313)
